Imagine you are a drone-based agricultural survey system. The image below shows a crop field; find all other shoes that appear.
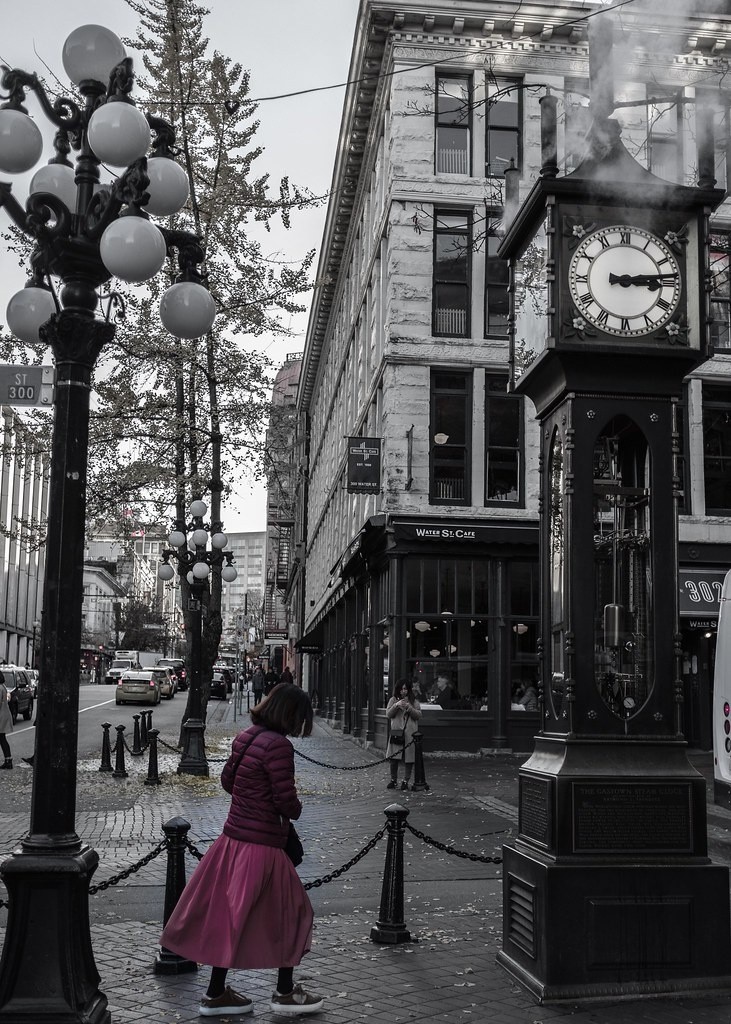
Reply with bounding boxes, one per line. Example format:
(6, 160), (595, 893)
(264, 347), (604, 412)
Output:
(21, 758), (34, 766)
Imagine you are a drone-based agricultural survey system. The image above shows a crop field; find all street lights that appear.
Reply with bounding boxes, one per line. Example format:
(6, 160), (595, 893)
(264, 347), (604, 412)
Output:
(0, 20), (213, 1024)
(157, 494), (238, 779)
(32, 619), (38, 668)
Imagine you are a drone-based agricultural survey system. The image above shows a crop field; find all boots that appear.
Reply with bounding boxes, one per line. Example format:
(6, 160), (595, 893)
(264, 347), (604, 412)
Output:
(0, 759), (13, 769)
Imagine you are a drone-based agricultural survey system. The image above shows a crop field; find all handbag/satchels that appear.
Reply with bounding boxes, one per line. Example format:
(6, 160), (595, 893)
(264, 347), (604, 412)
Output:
(284, 821), (304, 868)
(390, 729), (405, 745)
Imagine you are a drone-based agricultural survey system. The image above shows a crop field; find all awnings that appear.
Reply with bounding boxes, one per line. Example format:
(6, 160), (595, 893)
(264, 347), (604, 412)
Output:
(679, 568), (730, 632)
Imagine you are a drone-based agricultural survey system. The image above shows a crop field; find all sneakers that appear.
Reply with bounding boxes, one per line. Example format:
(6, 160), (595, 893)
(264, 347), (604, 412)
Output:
(270, 983), (324, 1012)
(387, 779), (397, 789)
(200, 985), (253, 1015)
(401, 781), (408, 790)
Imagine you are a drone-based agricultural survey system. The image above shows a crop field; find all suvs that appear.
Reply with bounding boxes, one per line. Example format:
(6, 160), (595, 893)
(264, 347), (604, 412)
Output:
(155, 659), (189, 691)
(0, 664), (34, 725)
(212, 666), (233, 692)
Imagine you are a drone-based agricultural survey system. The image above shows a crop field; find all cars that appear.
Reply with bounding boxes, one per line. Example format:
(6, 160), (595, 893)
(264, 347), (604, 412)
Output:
(142, 665), (179, 699)
(229, 663), (244, 682)
(211, 673), (229, 700)
(116, 670), (163, 706)
(26, 669), (39, 698)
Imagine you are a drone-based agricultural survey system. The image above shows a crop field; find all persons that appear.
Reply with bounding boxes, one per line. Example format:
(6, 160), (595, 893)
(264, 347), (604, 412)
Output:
(21, 720), (36, 765)
(428, 674), (458, 710)
(411, 676), (427, 702)
(514, 680), (536, 711)
(385, 678), (422, 790)
(255, 664), (265, 675)
(280, 667), (293, 683)
(253, 666), (279, 706)
(158, 682), (324, 1015)
(0, 671), (13, 769)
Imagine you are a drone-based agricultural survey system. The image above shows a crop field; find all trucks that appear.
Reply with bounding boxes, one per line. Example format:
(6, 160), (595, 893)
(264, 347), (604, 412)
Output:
(105, 650), (165, 685)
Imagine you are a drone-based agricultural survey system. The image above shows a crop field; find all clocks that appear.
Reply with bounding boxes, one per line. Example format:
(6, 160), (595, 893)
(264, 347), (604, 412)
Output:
(570, 225), (682, 337)
